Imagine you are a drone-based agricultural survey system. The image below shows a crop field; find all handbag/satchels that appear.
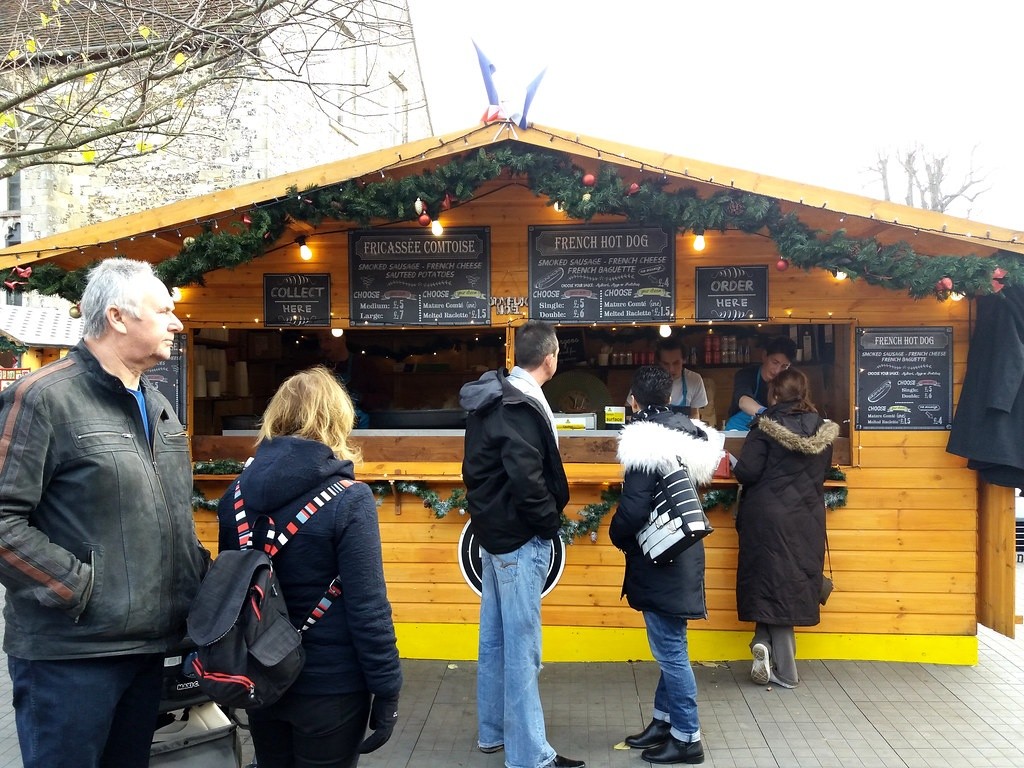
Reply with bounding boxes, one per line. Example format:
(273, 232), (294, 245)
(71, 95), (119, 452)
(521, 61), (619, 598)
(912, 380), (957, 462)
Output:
(632, 462), (714, 564)
(820, 575), (833, 606)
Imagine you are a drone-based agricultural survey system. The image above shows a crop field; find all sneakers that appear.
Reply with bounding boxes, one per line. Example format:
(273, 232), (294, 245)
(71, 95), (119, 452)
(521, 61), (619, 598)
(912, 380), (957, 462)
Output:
(767, 665), (796, 688)
(751, 644), (769, 686)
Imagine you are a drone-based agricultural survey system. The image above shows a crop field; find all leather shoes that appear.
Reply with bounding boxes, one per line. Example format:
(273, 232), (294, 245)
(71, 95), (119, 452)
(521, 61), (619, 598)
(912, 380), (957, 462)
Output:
(543, 755), (586, 768)
(641, 732), (705, 764)
(625, 717), (673, 748)
(479, 745), (503, 752)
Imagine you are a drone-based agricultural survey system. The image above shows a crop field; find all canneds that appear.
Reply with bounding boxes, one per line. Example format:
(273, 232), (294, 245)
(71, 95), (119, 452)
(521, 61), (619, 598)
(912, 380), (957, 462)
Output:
(610, 351), (656, 365)
(689, 334), (752, 365)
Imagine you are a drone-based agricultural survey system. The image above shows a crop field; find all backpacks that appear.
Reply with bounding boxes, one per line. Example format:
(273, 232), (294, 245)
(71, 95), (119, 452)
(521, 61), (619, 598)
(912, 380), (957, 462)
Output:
(179, 479), (364, 710)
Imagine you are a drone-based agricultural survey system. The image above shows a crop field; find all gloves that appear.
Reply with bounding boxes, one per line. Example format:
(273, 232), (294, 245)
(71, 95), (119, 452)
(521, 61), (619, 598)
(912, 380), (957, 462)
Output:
(359, 695), (398, 755)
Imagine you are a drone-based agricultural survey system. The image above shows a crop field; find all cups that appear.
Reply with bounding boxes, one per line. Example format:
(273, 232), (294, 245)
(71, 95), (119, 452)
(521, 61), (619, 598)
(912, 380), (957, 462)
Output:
(191, 329), (249, 399)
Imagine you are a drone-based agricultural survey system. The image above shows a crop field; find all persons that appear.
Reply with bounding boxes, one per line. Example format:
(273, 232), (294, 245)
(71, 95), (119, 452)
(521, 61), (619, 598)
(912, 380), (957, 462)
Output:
(218, 368), (402, 768)
(624, 341), (708, 420)
(725, 337), (797, 431)
(0, 259), (212, 767)
(608, 364), (723, 764)
(316, 329), (378, 430)
(458, 319), (585, 768)
(733, 368), (839, 687)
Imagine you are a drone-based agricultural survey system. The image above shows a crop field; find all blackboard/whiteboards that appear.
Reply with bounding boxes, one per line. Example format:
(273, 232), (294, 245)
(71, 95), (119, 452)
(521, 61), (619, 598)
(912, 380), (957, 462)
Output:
(263, 272), (331, 326)
(695, 265), (769, 322)
(143, 334), (187, 430)
(856, 326), (953, 430)
(527, 221), (676, 323)
(347, 227), (492, 325)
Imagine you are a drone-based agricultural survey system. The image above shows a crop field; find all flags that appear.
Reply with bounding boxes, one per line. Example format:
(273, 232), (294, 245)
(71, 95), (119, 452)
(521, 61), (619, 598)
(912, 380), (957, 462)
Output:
(472, 42), (505, 121)
(510, 69), (544, 129)
